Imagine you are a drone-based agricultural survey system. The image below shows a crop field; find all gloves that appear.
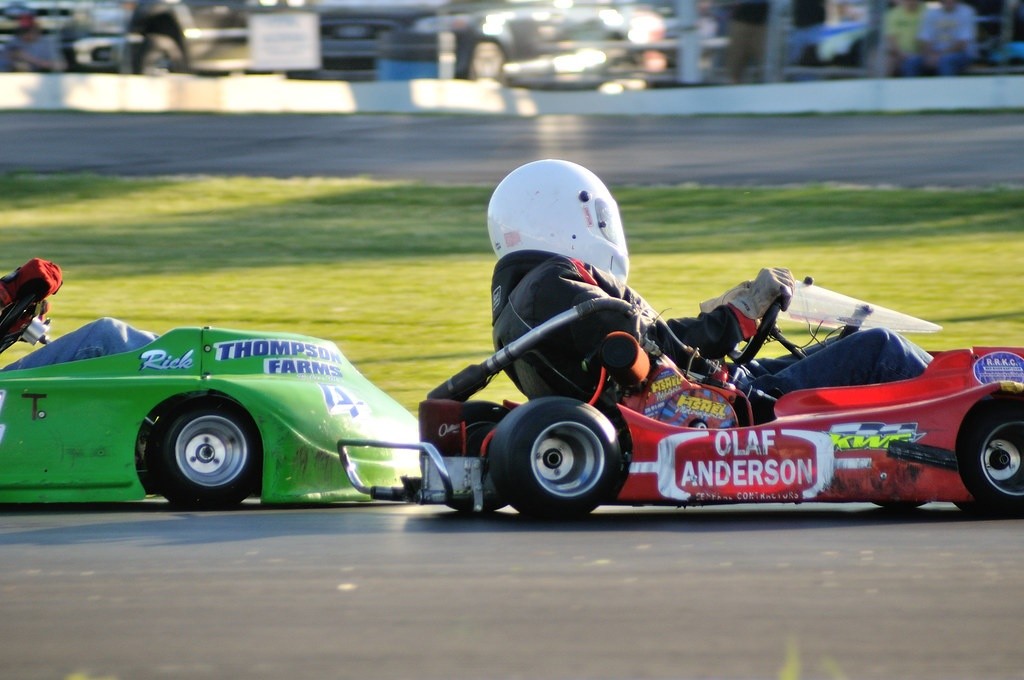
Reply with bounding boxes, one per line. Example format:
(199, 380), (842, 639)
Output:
(726, 266), (795, 320)
(699, 280), (754, 314)
(0, 257), (64, 305)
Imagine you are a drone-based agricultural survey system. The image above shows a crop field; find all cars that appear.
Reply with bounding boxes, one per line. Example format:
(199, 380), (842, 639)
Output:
(281, 0), (715, 84)
(55, 0), (255, 78)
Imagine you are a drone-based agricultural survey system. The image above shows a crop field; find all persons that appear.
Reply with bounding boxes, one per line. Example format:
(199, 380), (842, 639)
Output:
(455, 37), (517, 88)
(488, 159), (934, 427)
(881, 0), (980, 79)
(0, 258), (64, 324)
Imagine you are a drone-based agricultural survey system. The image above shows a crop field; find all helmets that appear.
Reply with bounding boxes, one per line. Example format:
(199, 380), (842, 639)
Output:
(487, 158), (628, 285)
